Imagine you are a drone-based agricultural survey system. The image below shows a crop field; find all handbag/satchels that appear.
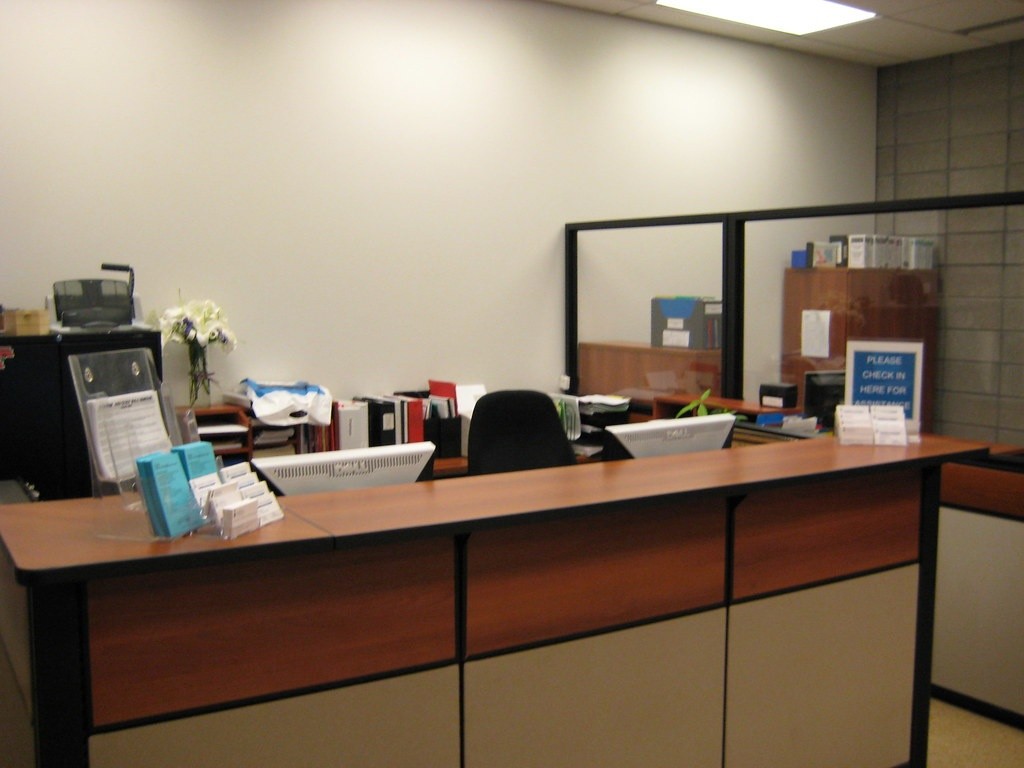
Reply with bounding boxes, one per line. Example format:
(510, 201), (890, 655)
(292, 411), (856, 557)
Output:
(238, 375), (333, 427)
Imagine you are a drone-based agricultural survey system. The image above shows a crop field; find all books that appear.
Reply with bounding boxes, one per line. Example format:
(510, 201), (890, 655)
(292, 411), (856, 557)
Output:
(423, 396), (455, 419)
(252, 428), (294, 445)
(213, 442), (241, 450)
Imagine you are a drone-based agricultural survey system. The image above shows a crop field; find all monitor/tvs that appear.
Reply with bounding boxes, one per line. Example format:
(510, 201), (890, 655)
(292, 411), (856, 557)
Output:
(602, 412), (737, 462)
(251, 440), (436, 497)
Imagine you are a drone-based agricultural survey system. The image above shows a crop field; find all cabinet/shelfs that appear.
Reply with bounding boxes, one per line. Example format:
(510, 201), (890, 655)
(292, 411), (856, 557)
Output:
(578, 341), (722, 397)
(175, 404), (253, 466)
(782, 268), (937, 432)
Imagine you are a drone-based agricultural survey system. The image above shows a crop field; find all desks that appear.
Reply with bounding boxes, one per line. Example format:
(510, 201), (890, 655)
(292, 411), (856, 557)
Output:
(433, 455), (601, 477)
(960, 440), (1024, 459)
(653, 393), (801, 425)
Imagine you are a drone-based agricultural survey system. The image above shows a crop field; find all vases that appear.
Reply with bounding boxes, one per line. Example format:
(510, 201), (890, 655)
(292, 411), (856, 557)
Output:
(188, 345), (211, 408)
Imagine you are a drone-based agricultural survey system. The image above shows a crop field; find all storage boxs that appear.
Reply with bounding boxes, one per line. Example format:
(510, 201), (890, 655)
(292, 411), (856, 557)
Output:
(3, 309), (50, 336)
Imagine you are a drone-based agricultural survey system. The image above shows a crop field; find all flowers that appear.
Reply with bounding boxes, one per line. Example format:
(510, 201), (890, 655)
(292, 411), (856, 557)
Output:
(148, 297), (238, 402)
